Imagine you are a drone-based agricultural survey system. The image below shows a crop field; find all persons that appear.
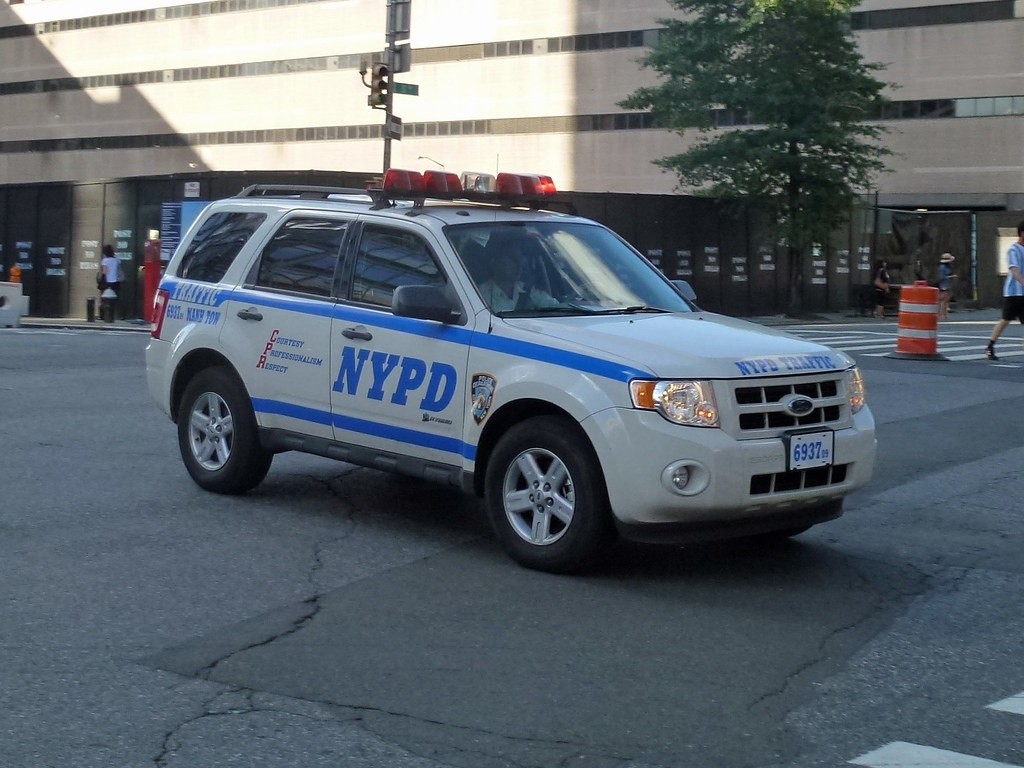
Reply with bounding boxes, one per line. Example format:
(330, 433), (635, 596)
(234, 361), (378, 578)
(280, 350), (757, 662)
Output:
(95, 245), (127, 322)
(936, 252), (960, 321)
(985, 220), (1024, 361)
(474, 237), (561, 313)
(872, 259), (890, 319)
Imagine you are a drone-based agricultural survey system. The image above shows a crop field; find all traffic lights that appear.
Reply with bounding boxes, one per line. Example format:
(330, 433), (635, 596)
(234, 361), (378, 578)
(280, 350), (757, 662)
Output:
(367, 50), (390, 108)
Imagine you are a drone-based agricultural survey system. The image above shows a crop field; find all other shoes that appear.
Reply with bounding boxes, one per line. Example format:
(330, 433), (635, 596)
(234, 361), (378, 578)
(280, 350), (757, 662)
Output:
(984, 346), (1000, 360)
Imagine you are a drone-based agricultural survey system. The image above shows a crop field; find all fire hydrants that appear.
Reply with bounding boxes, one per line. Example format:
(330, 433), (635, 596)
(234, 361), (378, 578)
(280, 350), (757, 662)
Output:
(99, 287), (119, 321)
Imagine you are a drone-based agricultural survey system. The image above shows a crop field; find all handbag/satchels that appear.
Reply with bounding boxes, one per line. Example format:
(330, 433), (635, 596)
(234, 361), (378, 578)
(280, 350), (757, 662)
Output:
(115, 257), (125, 282)
(874, 268), (888, 289)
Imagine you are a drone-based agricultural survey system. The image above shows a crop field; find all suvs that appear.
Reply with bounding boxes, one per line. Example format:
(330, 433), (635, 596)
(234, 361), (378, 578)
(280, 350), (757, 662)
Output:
(145, 162), (880, 576)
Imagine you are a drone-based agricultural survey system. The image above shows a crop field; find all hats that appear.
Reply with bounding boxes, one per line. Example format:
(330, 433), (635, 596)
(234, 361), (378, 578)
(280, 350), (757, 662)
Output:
(940, 253), (955, 263)
